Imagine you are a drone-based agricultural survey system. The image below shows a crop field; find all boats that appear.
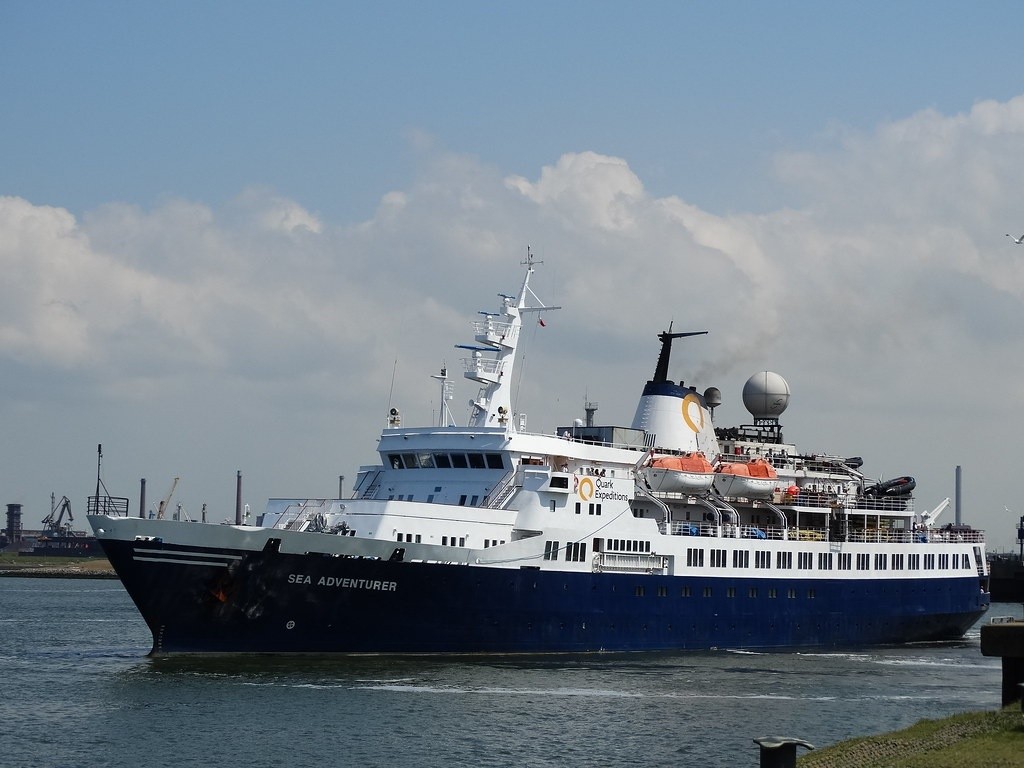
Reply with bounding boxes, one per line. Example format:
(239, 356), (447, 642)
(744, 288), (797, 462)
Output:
(86, 242), (991, 662)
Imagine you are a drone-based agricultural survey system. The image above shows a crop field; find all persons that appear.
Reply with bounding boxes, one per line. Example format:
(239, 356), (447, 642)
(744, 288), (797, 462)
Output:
(912, 521), (928, 543)
(945, 523), (952, 540)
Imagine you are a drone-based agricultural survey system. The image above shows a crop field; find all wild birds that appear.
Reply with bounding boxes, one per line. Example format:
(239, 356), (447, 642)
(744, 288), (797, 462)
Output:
(1005, 233), (1024, 247)
